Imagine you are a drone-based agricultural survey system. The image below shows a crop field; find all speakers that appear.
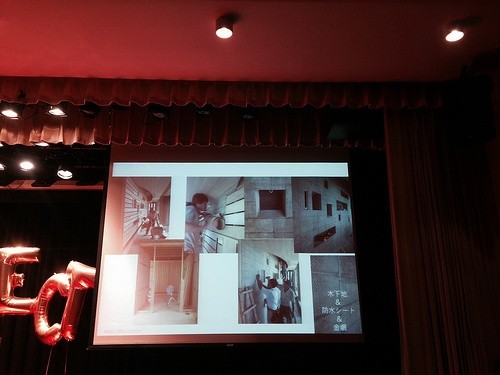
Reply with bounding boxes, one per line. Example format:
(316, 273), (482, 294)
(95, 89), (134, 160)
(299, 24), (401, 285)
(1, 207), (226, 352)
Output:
(437, 73), (496, 149)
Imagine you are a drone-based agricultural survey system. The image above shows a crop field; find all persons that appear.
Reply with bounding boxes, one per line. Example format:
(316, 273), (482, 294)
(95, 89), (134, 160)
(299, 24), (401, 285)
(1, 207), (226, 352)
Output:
(142, 207), (167, 240)
(256, 273), (282, 324)
(185, 176), (223, 254)
(266, 276), (295, 324)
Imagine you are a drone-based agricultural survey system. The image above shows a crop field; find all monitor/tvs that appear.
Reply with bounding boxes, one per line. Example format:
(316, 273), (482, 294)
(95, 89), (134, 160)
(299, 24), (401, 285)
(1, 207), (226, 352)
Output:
(87, 144), (367, 347)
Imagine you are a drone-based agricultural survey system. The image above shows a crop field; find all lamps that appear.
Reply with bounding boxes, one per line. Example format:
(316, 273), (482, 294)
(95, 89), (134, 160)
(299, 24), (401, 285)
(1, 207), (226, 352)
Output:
(1, 103), (253, 181)
(216, 16), (233, 39)
(445, 22), (464, 43)
